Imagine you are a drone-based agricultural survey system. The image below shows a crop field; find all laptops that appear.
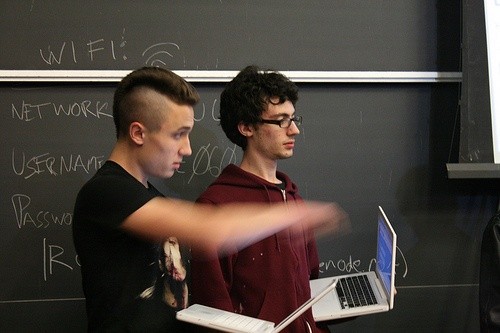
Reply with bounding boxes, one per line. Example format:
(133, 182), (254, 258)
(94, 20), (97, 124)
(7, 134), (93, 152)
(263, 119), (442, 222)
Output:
(176, 278), (337, 333)
(309, 205), (398, 322)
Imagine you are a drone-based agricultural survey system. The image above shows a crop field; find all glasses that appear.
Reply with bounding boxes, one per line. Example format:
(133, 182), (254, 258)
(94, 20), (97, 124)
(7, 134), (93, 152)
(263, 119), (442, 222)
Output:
(254, 116), (302, 128)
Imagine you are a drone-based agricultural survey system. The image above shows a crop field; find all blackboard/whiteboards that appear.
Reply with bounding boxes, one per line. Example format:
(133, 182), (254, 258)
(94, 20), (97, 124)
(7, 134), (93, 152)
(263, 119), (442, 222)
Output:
(0, 81), (498, 332)
(2, 0), (464, 73)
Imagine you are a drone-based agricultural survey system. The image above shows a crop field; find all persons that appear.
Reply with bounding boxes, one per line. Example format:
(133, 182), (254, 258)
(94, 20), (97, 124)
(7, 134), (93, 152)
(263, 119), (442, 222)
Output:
(190, 65), (332, 332)
(71, 66), (354, 333)
(480, 206), (500, 333)
